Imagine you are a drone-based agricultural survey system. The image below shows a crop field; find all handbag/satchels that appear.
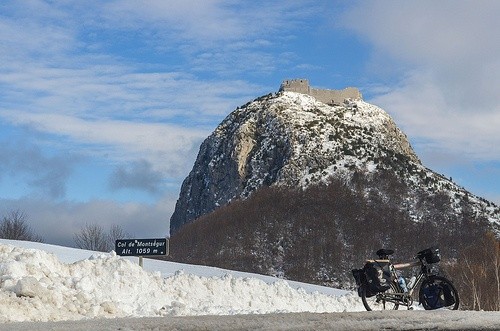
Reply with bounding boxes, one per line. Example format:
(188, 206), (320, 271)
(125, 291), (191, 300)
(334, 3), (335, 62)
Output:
(423, 247), (441, 264)
(351, 267), (379, 297)
(362, 261), (393, 292)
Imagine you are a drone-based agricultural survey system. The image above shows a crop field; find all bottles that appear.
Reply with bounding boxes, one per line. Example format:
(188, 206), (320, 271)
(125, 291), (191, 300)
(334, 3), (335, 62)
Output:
(398, 275), (409, 293)
(406, 274), (417, 290)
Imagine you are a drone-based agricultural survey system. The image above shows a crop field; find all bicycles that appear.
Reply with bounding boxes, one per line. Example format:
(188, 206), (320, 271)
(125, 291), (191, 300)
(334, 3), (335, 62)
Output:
(360, 246), (459, 312)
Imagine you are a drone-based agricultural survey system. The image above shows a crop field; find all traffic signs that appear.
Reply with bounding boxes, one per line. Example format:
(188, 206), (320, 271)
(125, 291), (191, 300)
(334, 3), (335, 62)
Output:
(114, 236), (169, 258)
